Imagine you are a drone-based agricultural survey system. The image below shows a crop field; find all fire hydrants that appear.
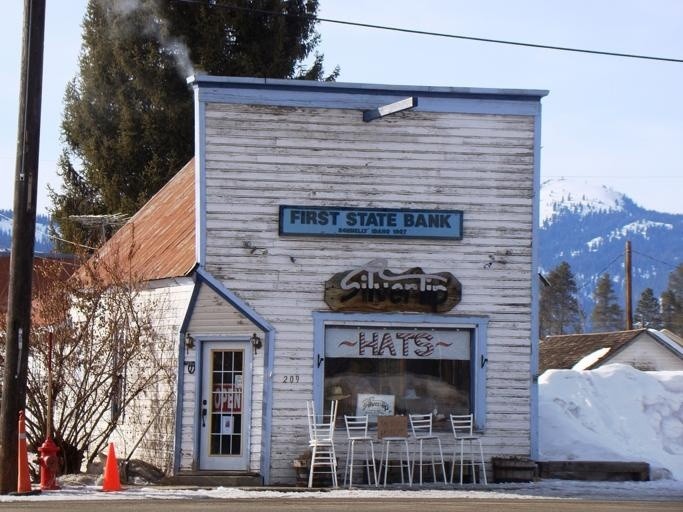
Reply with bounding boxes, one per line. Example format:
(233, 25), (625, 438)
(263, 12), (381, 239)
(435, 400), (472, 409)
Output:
(38, 435), (64, 490)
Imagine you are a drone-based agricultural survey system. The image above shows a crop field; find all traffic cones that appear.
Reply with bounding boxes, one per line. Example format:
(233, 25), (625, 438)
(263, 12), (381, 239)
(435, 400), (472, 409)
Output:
(8, 412), (41, 496)
(102, 443), (126, 492)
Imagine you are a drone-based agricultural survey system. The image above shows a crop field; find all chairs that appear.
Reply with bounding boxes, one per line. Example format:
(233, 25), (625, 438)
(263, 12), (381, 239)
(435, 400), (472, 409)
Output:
(305, 399), (488, 490)
